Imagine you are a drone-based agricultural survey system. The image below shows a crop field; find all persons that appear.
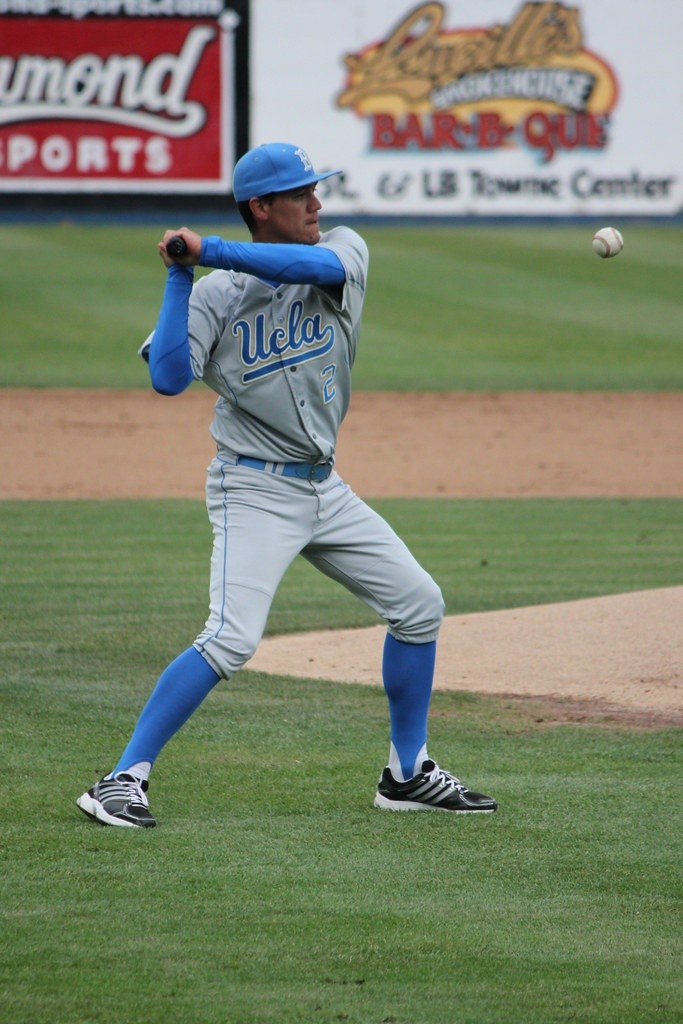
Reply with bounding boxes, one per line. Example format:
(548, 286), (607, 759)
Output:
(73, 142), (500, 830)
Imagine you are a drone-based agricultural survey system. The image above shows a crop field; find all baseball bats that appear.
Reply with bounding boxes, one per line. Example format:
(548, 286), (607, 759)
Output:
(166, 237), (188, 260)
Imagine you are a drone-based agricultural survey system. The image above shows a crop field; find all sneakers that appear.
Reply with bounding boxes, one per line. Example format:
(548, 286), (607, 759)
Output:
(76, 773), (156, 829)
(372, 758), (497, 814)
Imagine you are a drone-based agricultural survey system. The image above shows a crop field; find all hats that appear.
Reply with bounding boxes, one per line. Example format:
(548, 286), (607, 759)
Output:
(230, 142), (342, 202)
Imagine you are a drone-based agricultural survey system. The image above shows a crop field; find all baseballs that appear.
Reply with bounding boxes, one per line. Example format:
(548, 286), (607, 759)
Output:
(591, 227), (626, 260)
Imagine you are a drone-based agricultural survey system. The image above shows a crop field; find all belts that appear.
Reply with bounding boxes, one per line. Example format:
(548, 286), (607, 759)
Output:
(237, 456), (331, 484)
